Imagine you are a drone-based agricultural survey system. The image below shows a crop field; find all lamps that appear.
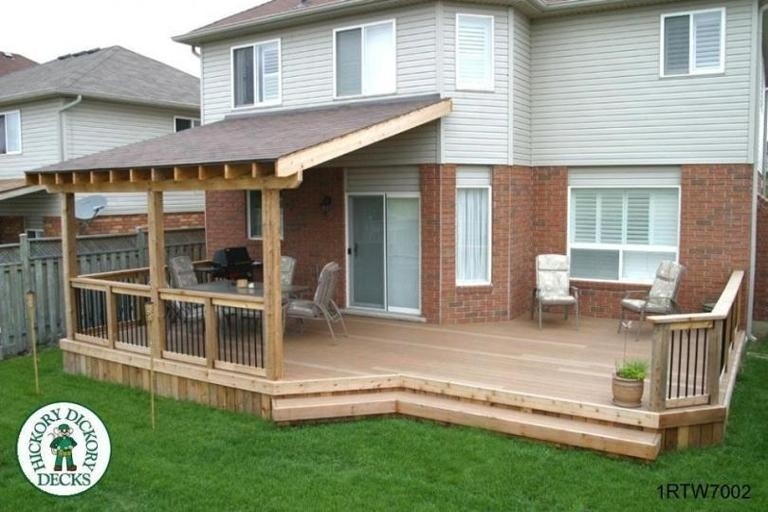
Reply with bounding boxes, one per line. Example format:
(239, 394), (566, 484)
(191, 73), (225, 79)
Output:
(319, 195), (332, 218)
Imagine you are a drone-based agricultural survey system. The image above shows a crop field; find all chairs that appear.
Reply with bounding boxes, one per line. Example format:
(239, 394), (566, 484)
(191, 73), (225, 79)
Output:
(616, 260), (687, 342)
(145, 255), (348, 345)
(531, 253), (580, 330)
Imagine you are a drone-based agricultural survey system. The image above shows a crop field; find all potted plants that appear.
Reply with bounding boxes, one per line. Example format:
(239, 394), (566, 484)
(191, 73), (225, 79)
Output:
(611, 356), (651, 408)
